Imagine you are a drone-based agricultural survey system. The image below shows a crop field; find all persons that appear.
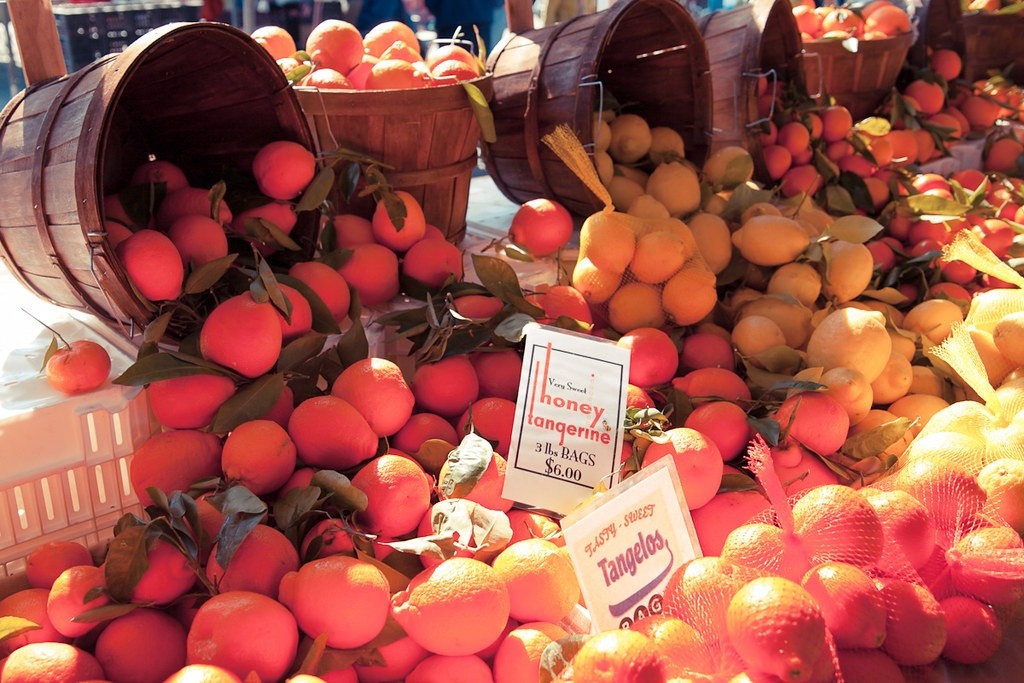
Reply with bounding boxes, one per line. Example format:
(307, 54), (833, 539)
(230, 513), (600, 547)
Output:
(424, 0), (503, 62)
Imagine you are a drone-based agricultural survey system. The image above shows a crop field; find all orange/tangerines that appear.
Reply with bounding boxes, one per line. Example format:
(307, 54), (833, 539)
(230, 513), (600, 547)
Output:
(1, 0), (1024, 680)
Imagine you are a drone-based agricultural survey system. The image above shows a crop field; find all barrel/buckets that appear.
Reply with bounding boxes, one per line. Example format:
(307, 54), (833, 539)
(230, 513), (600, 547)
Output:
(4, 2), (1022, 350)
